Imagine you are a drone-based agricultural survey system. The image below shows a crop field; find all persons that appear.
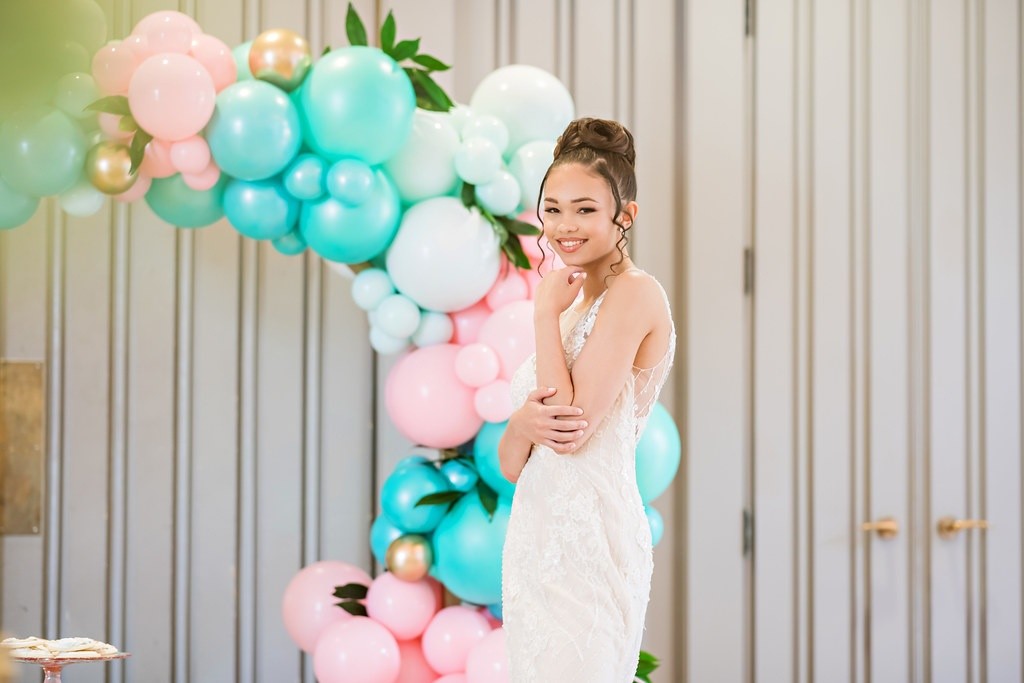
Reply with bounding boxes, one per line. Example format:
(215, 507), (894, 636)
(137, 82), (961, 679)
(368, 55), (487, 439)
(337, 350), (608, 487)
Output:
(498, 118), (677, 683)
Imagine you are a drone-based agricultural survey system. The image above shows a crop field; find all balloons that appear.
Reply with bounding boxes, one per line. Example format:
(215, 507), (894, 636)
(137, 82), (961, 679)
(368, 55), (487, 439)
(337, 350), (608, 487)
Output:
(637, 405), (681, 546)
(0, 0), (574, 683)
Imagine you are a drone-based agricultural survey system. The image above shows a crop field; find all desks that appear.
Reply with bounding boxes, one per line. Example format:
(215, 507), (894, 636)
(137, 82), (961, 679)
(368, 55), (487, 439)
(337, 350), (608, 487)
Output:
(6, 647), (132, 683)
